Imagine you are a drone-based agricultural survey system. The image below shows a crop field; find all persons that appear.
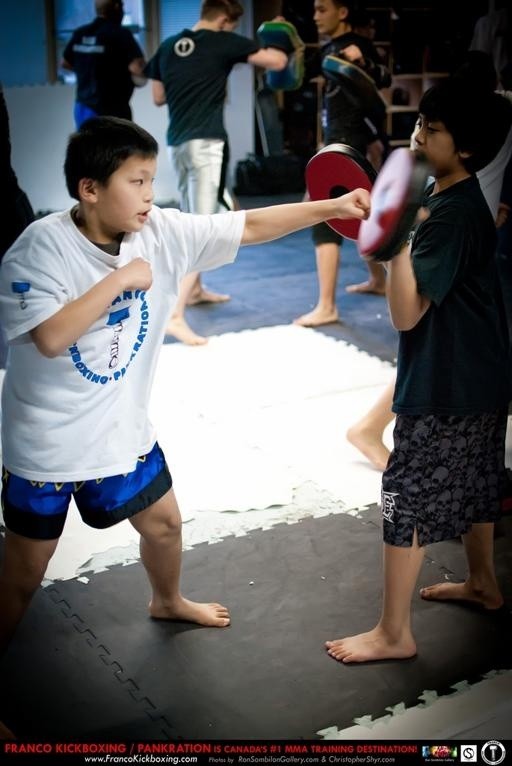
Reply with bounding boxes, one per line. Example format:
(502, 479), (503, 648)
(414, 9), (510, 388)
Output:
(273, 0), (388, 327)
(139, 1), (291, 348)
(343, 48), (512, 470)
(58, 0), (146, 132)
(323, 72), (512, 666)
(0, 114), (374, 648)
(1, 78), (36, 264)
